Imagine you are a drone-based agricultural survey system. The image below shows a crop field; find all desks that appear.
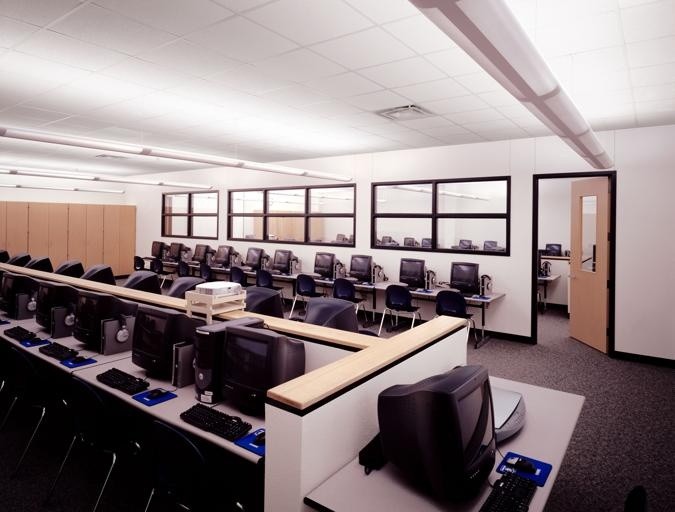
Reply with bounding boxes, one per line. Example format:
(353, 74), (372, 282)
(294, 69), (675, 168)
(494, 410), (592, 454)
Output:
(304, 373), (586, 510)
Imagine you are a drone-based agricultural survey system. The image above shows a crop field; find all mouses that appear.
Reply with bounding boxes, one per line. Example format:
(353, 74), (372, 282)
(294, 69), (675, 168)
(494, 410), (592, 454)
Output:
(515, 460), (536, 474)
(72, 356), (86, 364)
(150, 390), (163, 399)
(254, 431), (265, 446)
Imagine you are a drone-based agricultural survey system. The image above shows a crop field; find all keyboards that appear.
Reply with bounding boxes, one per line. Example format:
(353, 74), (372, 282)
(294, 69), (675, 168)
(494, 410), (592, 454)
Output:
(39, 342), (79, 361)
(478, 471), (538, 512)
(96, 368), (150, 396)
(179, 403), (254, 443)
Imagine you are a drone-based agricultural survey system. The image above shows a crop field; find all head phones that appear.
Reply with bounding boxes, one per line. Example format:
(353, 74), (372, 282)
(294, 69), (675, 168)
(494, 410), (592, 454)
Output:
(481, 275), (493, 290)
(542, 261), (552, 272)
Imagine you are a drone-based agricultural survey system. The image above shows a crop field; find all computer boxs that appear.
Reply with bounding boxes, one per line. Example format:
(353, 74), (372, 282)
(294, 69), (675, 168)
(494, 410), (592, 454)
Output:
(171, 341), (194, 388)
(194, 316), (265, 404)
(544, 263), (551, 276)
(480, 278), (492, 296)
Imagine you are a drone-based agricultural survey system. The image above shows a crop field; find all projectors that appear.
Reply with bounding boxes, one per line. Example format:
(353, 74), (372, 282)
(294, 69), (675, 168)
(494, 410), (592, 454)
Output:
(196, 281), (242, 297)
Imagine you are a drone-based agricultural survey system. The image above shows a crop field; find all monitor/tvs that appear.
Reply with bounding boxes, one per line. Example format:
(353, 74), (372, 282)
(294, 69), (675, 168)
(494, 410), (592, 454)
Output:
(377, 364), (497, 505)
(132, 309), (196, 381)
(336, 234), (562, 257)
(225, 326), (307, 421)
